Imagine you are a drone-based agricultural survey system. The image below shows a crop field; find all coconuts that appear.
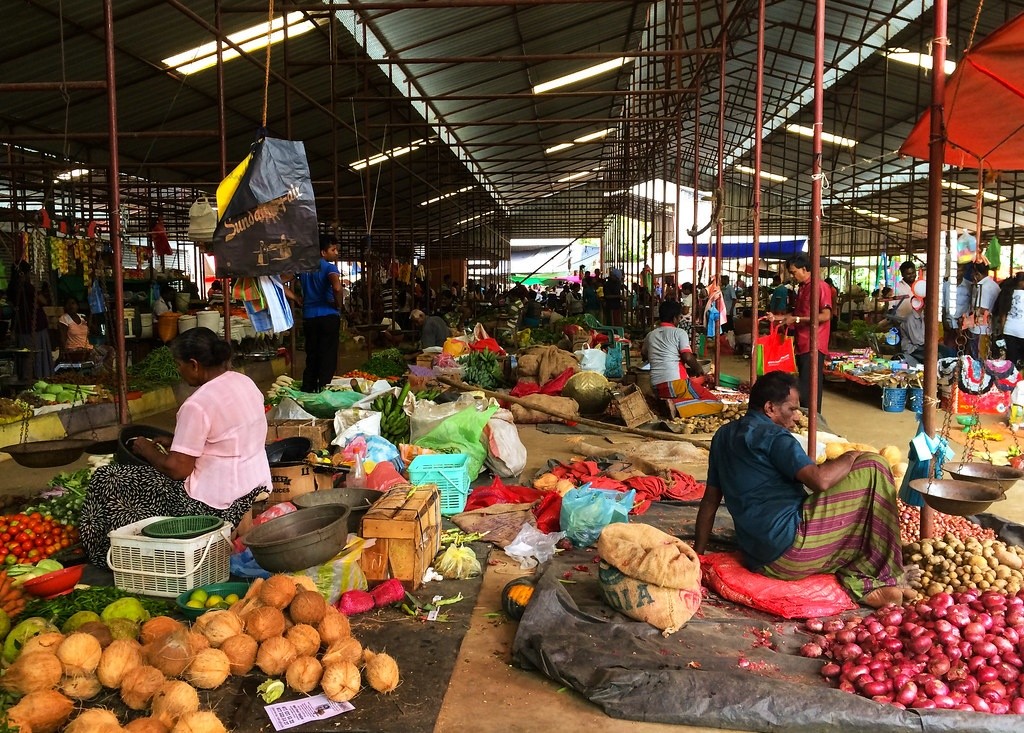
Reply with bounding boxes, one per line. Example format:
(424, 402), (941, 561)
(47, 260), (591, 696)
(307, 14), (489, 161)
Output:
(193, 574), (401, 702)
(0, 596), (231, 733)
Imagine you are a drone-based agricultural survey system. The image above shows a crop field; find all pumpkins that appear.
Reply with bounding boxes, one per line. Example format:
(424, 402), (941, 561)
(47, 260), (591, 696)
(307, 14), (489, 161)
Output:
(501, 576), (539, 619)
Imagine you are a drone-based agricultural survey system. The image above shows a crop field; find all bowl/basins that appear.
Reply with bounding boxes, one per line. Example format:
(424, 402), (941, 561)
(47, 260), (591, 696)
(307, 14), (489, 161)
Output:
(719, 374), (742, 389)
(685, 358), (712, 376)
(117, 424), (174, 465)
(292, 487), (385, 532)
(242, 502), (353, 574)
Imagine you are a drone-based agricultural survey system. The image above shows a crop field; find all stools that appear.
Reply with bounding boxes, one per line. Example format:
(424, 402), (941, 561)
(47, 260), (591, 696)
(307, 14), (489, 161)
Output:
(365, 485), (442, 592)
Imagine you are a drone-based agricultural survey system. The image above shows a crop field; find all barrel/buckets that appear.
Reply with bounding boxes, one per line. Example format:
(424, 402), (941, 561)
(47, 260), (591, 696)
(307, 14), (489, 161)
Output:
(140, 313), (153, 339)
(176, 293), (190, 312)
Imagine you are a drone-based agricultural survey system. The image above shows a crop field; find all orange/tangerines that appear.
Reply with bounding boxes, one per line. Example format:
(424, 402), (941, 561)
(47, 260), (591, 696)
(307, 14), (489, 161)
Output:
(185, 588), (243, 608)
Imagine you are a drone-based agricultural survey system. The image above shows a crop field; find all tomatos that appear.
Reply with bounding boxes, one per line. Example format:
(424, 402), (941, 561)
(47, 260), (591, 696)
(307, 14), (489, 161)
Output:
(0, 511), (81, 565)
(343, 369), (400, 382)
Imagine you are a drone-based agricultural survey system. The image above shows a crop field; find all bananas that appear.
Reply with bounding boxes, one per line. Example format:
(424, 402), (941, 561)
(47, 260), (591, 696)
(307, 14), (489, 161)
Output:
(455, 346), (499, 390)
(371, 386), (439, 447)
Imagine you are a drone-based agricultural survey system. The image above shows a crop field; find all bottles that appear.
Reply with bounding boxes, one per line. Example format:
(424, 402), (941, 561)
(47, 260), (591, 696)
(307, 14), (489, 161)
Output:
(345, 449), (367, 489)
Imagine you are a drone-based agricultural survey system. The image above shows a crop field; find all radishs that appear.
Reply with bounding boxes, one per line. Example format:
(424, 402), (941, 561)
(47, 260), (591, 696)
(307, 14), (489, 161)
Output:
(266, 376), (294, 399)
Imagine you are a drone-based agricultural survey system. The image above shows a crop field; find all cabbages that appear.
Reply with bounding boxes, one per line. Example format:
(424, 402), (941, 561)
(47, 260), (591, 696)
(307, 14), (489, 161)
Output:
(30, 383), (75, 403)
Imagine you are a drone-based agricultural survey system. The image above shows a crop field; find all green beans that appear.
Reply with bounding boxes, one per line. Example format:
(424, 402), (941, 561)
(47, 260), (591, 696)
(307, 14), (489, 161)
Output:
(125, 345), (181, 390)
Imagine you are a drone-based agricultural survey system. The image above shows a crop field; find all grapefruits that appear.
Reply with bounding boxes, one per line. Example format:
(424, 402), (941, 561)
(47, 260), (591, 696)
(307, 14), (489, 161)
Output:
(813, 441), (908, 491)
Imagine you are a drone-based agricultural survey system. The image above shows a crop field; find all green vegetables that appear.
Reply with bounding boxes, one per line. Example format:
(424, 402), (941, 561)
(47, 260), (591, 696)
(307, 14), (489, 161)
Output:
(361, 347), (409, 378)
(531, 313), (589, 344)
(47, 466), (89, 510)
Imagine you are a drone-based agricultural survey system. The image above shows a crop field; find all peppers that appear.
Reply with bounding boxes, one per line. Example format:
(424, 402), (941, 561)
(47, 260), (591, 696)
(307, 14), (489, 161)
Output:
(9, 583), (182, 633)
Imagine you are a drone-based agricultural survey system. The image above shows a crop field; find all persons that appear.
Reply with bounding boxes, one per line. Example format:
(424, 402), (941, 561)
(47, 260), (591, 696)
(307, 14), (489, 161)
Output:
(633, 276), (709, 330)
(721, 275), (737, 335)
(872, 261), (916, 354)
(734, 274), (746, 289)
(342, 258), (630, 331)
(280, 235), (343, 392)
(6, 261), (54, 395)
(758, 251), (832, 415)
(208, 277), (222, 300)
(941, 261), (1024, 371)
(403, 310), (450, 365)
(692, 370), (916, 610)
(57, 294), (114, 373)
(899, 296), (957, 364)
(80, 326), (272, 561)
(824, 278), (839, 349)
(228, 277), (238, 296)
(152, 287), (174, 325)
(771, 275), (800, 315)
(639, 300), (724, 420)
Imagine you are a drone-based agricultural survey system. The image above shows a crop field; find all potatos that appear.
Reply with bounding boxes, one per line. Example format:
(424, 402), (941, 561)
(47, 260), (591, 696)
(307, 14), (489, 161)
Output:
(672, 402), (810, 436)
(903, 538), (1024, 605)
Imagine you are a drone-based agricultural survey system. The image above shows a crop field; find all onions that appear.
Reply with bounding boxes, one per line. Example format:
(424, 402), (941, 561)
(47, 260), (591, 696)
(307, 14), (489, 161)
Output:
(801, 588), (1024, 717)
(896, 498), (999, 545)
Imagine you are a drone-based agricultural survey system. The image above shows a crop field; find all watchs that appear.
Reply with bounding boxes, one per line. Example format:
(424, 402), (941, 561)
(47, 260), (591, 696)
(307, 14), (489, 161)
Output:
(795, 316), (800, 324)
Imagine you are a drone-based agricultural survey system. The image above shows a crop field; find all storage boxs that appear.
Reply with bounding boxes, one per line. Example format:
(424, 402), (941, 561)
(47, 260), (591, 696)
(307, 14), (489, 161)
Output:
(267, 464), (333, 504)
(106, 515), (233, 599)
(406, 453), (470, 514)
(265, 418), (333, 454)
(615, 385), (656, 429)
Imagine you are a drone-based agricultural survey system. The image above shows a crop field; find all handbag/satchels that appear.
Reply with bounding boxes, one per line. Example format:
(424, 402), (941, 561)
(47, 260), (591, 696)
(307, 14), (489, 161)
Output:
(755, 317), (800, 378)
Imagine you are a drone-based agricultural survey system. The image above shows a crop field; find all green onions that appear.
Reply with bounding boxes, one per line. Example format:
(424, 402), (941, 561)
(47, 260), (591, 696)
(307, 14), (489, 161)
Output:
(61, 384), (109, 397)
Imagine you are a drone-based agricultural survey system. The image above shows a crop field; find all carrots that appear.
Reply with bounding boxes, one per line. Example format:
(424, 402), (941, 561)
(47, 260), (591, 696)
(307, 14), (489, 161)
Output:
(0, 567), (29, 618)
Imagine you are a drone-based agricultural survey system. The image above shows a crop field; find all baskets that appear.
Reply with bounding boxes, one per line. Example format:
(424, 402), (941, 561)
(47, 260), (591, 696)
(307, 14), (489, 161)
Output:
(883, 388), (907, 413)
(909, 389), (925, 412)
(107, 516), (233, 598)
(407, 453), (471, 513)
